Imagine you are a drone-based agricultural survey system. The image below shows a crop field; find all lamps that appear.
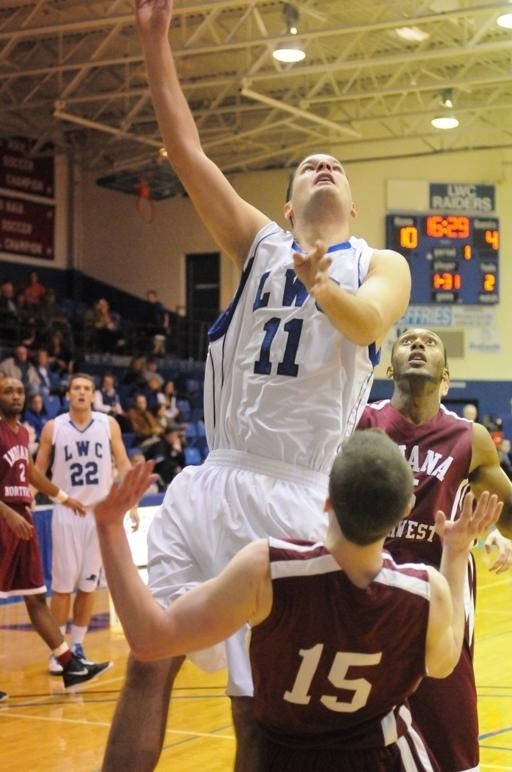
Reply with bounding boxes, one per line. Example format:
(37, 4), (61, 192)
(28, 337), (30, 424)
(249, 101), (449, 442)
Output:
(273, 5), (307, 64)
(431, 89), (460, 130)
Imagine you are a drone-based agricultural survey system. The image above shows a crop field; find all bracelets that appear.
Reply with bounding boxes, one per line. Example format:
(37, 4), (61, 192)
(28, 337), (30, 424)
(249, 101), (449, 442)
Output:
(48, 488), (69, 504)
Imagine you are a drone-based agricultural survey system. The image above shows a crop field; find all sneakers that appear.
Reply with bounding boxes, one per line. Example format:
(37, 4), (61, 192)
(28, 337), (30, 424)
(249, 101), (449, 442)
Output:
(49, 651), (64, 675)
(71, 643), (86, 662)
(63, 660), (113, 688)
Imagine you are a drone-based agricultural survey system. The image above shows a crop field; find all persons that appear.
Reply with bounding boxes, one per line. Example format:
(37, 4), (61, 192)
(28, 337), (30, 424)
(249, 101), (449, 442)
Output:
(95, 425), (505, 772)
(357, 328), (512, 772)
(463, 401), (512, 575)
(100, 1), (411, 772)
(1, 375), (114, 704)
(1, 271), (186, 495)
(25, 372), (139, 675)
